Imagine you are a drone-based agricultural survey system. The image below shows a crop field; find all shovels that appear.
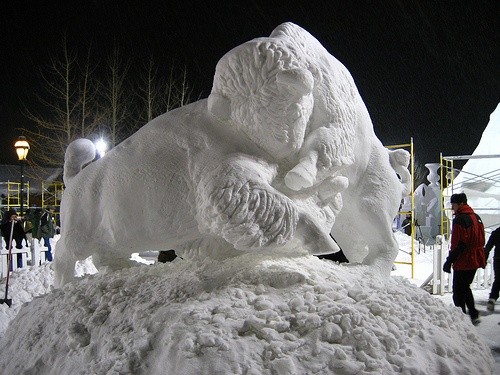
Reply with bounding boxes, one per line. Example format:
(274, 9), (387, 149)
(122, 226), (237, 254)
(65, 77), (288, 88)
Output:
(0, 219), (15, 308)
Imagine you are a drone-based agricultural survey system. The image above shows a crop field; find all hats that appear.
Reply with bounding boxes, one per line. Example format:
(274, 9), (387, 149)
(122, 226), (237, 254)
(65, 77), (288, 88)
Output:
(450, 193), (467, 202)
(8, 209), (18, 217)
(46, 205), (56, 209)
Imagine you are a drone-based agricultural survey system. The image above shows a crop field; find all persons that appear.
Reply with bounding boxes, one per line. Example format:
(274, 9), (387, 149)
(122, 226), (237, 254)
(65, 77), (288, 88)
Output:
(443, 192), (488, 326)
(402, 210), (420, 234)
(30, 204), (55, 261)
(0, 208), (28, 271)
(484, 226), (500, 312)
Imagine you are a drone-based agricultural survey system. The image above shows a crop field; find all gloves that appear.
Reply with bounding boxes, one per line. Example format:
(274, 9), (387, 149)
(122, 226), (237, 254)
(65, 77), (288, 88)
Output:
(443, 261), (451, 273)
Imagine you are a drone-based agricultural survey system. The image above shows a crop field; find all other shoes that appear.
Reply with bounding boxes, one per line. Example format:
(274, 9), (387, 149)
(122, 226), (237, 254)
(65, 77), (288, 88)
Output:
(487, 301), (494, 312)
(472, 319), (481, 326)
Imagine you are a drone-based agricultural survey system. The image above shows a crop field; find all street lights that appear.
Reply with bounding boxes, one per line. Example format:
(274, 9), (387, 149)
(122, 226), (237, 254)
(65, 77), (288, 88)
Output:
(14, 133), (30, 237)
